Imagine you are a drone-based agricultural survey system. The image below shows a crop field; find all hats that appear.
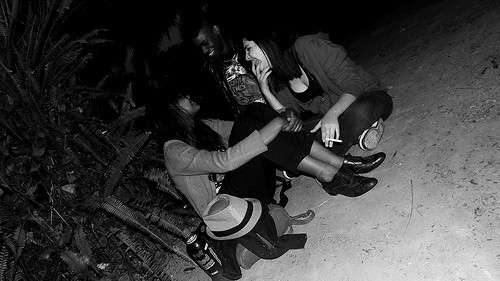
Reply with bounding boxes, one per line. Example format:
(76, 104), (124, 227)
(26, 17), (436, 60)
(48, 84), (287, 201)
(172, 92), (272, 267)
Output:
(200, 192), (262, 242)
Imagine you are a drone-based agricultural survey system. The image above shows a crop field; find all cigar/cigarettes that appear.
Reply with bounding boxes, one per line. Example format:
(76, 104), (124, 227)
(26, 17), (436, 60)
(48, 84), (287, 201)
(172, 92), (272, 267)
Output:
(328, 138), (343, 143)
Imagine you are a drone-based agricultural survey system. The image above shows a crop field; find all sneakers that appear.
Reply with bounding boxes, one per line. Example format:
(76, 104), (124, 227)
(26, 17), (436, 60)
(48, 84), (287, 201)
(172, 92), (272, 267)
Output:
(359, 117), (387, 152)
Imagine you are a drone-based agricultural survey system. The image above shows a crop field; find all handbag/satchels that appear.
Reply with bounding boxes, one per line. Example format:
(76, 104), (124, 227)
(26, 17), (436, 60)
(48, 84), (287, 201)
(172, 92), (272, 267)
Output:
(231, 204), (315, 270)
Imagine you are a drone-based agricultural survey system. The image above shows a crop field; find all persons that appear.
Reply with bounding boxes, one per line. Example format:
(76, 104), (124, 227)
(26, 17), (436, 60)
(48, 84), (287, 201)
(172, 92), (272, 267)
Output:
(239, 21), (395, 154)
(146, 86), (388, 227)
(180, 15), (269, 105)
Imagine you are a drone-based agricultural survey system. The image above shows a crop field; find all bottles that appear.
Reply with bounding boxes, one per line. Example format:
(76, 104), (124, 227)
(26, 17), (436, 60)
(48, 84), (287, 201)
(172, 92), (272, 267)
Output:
(182, 227), (222, 278)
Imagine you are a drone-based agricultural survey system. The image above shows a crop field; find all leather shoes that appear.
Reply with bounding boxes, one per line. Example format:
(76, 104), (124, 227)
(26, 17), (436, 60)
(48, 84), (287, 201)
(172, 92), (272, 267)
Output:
(338, 151), (386, 174)
(319, 169), (379, 199)
